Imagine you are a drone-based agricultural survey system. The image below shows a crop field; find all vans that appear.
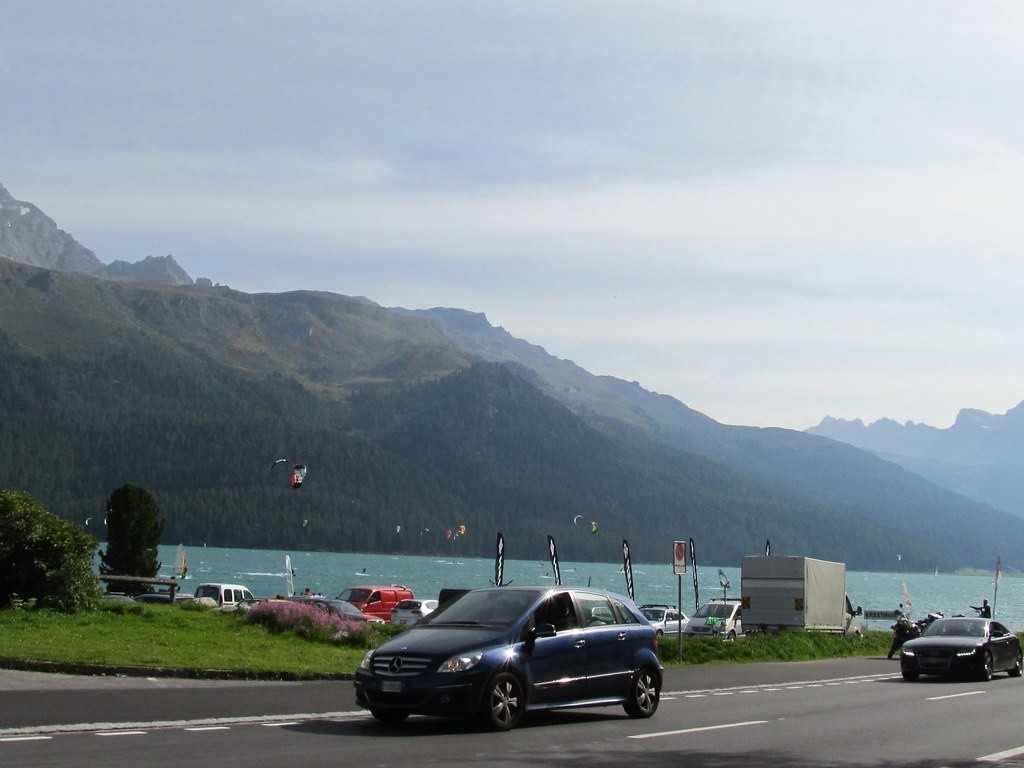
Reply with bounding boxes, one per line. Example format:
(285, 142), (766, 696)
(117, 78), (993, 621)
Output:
(683, 599), (744, 643)
(334, 586), (414, 626)
(193, 581), (256, 608)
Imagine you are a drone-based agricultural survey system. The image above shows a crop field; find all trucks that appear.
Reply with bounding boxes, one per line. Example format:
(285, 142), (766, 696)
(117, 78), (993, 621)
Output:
(741, 555), (865, 645)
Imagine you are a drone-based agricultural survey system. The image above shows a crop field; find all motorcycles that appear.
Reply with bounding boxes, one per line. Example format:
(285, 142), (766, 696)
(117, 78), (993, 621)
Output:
(887, 604), (946, 661)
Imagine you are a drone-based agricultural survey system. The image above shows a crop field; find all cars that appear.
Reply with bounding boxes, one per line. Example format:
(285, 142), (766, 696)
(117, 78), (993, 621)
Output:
(295, 598), (387, 626)
(901, 616), (1023, 681)
(389, 597), (442, 629)
(634, 602), (691, 638)
(133, 593), (195, 606)
(351, 585), (664, 731)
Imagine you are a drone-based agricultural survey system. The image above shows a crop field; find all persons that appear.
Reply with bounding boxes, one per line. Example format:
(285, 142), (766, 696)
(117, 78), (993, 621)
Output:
(969, 599), (991, 619)
